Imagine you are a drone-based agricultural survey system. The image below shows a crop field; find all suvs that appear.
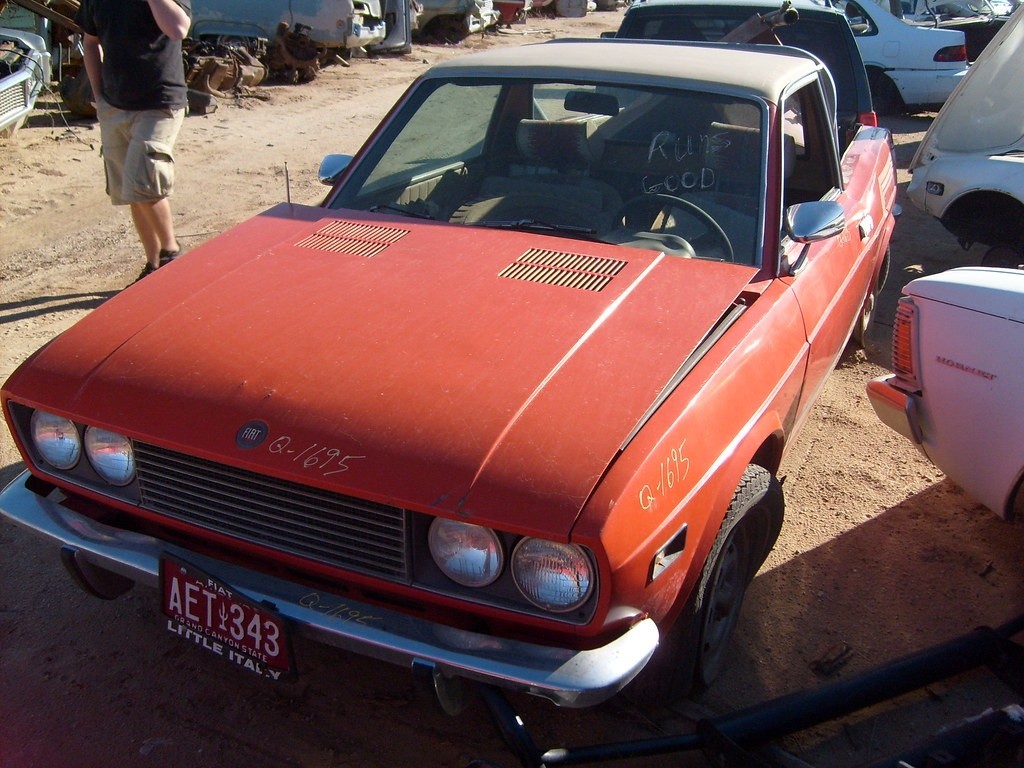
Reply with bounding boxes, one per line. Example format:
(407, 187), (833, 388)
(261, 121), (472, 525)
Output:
(592, 0), (878, 166)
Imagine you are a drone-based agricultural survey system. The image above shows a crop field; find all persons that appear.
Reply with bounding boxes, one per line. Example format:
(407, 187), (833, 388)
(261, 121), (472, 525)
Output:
(76, 0), (192, 289)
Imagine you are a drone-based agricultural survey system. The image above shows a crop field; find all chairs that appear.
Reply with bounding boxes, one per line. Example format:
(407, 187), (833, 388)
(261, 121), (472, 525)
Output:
(502, 119), (621, 229)
(640, 122), (794, 267)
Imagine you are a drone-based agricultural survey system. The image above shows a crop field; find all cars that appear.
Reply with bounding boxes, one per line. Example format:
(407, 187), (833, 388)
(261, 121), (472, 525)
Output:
(0, 0), (904, 716)
(809, 0), (972, 117)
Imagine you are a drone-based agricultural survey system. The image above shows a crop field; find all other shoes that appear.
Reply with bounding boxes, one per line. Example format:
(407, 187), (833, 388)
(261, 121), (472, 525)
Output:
(125, 262), (157, 290)
(159, 238), (182, 269)
